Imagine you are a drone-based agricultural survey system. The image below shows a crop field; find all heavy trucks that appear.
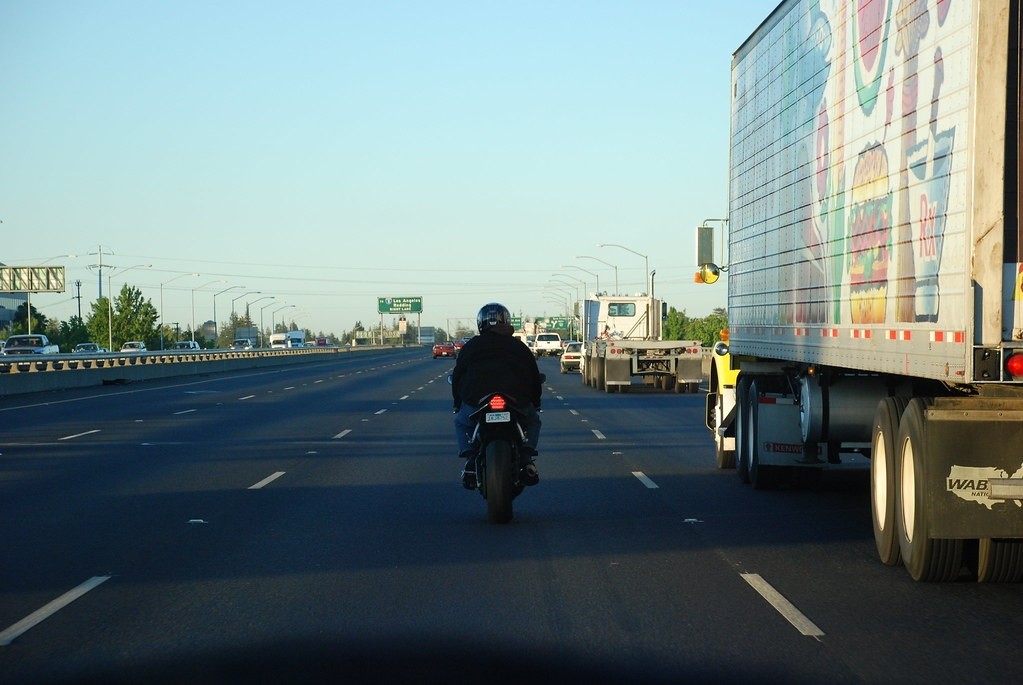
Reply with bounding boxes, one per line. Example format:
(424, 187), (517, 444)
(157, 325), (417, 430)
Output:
(696, 1), (1023, 588)
(574, 293), (704, 395)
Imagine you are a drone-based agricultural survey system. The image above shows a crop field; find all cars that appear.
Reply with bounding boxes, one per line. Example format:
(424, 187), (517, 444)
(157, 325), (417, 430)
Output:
(0, 341), (6, 354)
(454, 337), (473, 353)
(305, 343), (314, 348)
(525, 332), (577, 361)
(558, 342), (583, 374)
(432, 340), (457, 360)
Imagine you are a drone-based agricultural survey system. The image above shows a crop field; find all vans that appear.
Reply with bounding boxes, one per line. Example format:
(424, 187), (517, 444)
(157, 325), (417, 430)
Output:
(119, 341), (148, 352)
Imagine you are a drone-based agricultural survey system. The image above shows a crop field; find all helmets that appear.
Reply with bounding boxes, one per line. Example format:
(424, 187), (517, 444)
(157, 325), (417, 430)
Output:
(477, 303), (511, 334)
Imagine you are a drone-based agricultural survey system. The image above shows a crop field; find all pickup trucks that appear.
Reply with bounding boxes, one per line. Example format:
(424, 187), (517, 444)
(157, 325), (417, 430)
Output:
(72, 343), (110, 354)
(5, 334), (60, 354)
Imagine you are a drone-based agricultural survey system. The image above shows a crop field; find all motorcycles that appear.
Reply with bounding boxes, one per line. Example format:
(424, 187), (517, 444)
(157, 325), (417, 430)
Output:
(447, 368), (546, 525)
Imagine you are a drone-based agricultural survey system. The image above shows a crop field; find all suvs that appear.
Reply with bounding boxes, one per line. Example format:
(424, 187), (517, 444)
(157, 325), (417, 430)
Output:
(167, 341), (200, 350)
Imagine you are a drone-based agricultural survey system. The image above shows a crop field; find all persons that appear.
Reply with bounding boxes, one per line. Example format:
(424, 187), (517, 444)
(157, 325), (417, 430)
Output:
(452, 303), (542, 491)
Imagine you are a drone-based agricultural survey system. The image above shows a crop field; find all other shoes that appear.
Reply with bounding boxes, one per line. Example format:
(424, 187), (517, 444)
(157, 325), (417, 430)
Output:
(519, 459), (539, 486)
(461, 457), (477, 490)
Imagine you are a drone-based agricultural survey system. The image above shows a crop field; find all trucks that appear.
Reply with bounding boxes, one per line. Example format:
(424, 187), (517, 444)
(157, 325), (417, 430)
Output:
(315, 337), (335, 348)
(229, 327), (259, 350)
(287, 330), (306, 349)
(268, 333), (292, 349)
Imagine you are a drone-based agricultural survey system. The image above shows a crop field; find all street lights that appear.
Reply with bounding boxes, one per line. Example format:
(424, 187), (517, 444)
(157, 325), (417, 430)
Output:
(231, 291), (263, 323)
(541, 272), (587, 317)
(594, 243), (649, 295)
(191, 278), (228, 343)
(576, 254), (618, 296)
(247, 296), (275, 322)
(562, 264), (599, 296)
(213, 285), (247, 340)
(27, 254), (79, 337)
(108, 264), (153, 352)
(272, 305), (296, 334)
(261, 301), (287, 348)
(160, 273), (201, 350)
